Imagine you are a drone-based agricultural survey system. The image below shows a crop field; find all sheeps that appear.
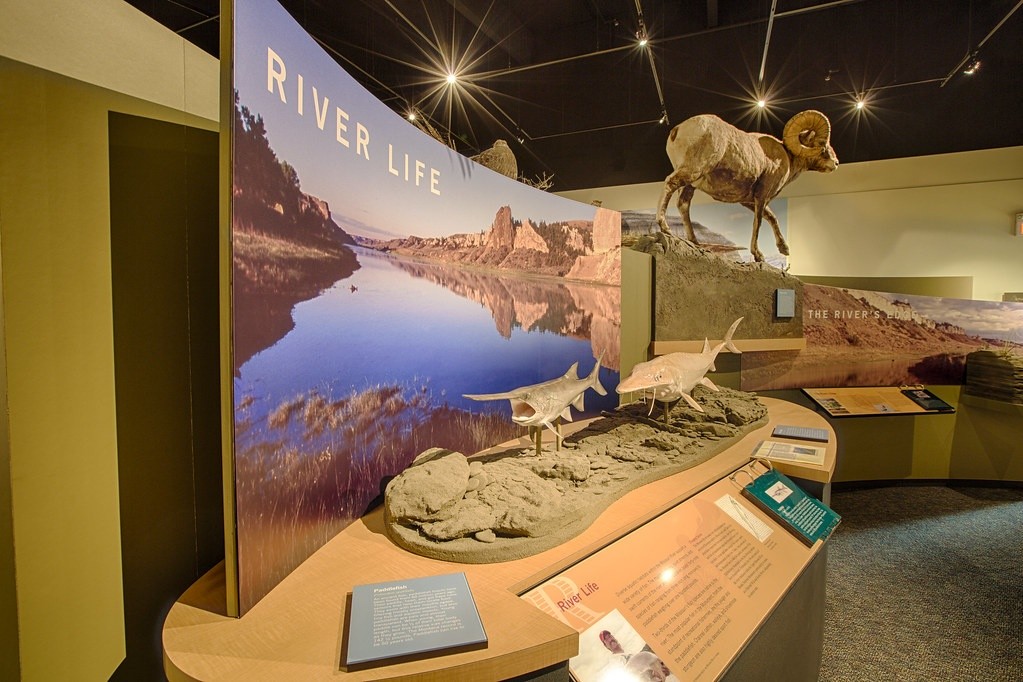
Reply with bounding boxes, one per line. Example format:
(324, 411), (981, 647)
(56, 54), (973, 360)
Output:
(655, 108), (840, 264)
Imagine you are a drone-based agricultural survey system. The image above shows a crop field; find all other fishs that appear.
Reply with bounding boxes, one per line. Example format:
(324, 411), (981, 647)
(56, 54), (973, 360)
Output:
(615, 317), (749, 416)
(460, 347), (609, 440)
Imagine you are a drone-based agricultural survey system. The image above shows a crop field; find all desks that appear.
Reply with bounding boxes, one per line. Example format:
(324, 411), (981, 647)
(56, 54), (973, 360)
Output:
(163, 398), (840, 682)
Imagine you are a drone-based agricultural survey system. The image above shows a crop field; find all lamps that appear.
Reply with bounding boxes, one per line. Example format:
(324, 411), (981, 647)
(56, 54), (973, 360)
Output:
(633, 25), (648, 46)
(658, 110), (666, 124)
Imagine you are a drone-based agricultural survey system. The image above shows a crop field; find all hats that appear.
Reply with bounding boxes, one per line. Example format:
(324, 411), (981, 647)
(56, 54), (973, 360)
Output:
(599, 630), (610, 641)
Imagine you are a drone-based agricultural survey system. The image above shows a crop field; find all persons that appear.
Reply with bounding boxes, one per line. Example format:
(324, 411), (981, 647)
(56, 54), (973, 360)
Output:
(600, 630), (635, 667)
